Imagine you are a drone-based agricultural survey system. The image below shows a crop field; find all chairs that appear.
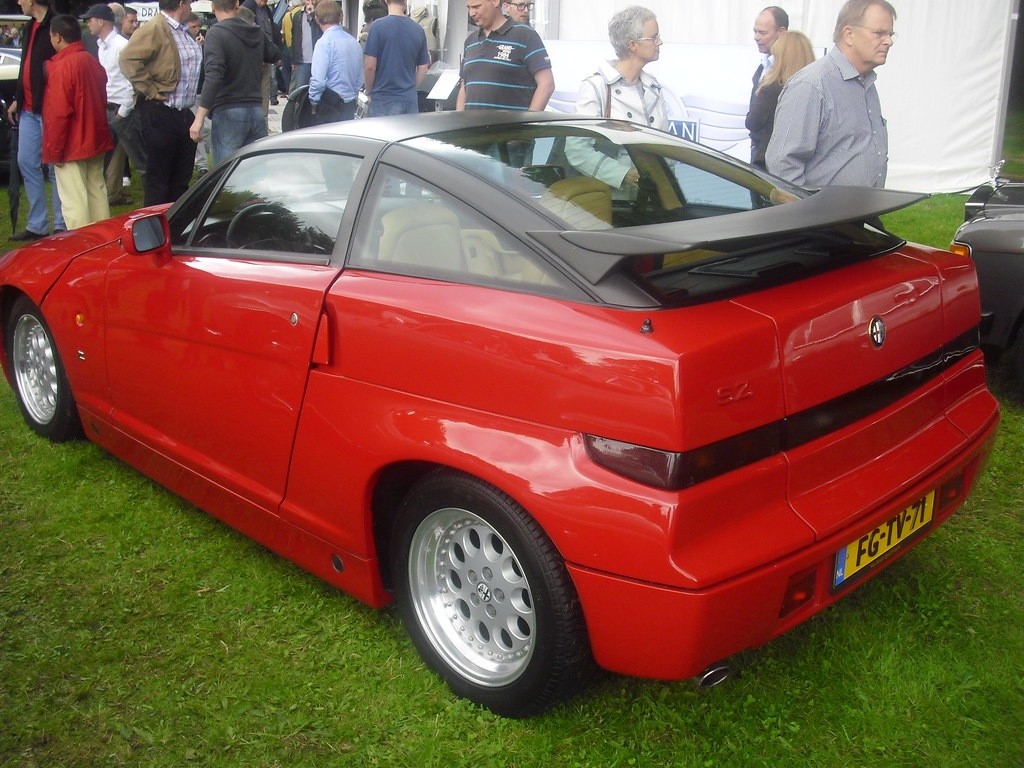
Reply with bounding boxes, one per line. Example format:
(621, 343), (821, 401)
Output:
(539, 175), (612, 230)
(378, 205), (463, 271)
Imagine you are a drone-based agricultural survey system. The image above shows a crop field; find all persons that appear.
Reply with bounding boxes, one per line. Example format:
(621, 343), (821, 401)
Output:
(0, 0), (430, 241)
(564, 6), (669, 200)
(456, 0), (556, 169)
(746, 0), (898, 212)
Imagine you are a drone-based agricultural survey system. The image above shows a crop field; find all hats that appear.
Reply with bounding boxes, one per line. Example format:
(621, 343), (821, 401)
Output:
(79, 4), (115, 20)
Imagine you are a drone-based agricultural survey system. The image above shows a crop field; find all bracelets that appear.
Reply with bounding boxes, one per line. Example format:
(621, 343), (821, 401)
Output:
(363, 89), (369, 96)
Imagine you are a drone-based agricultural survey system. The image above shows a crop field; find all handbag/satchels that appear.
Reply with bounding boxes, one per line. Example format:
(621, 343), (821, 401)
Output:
(542, 72), (619, 179)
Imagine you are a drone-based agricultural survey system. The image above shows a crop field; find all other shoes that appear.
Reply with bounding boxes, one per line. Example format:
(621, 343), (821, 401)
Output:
(9, 230), (49, 242)
(271, 98), (279, 105)
(54, 230), (64, 234)
(121, 190), (132, 196)
(109, 198), (132, 206)
(198, 166), (208, 179)
(123, 176), (133, 186)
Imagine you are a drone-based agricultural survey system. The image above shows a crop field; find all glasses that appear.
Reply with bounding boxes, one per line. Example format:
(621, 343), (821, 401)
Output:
(638, 34), (660, 44)
(509, 3), (534, 10)
(851, 24), (895, 38)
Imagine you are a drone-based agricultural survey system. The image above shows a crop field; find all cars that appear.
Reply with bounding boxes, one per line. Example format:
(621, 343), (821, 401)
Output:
(948, 158), (1024, 379)
(0, 108), (1003, 722)
